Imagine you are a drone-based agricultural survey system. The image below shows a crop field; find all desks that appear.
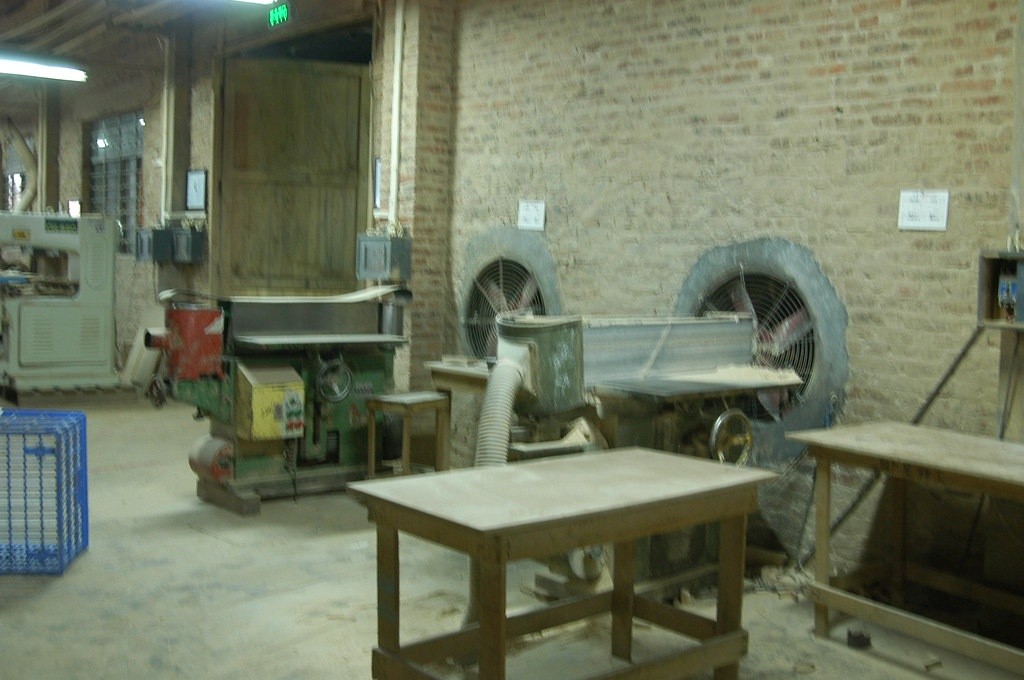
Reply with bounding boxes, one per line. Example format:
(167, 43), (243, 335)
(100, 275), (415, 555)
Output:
(345, 444), (781, 680)
(784, 420), (1024, 676)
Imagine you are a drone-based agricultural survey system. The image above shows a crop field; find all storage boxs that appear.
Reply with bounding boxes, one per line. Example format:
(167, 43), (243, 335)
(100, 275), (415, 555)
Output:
(0, 408), (89, 576)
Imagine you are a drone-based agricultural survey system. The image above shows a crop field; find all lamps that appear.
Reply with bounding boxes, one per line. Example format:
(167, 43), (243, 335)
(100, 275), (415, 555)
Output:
(0, 53), (89, 82)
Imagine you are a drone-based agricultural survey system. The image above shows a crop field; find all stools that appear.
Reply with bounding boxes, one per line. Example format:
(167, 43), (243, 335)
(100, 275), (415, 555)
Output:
(365, 391), (450, 521)
